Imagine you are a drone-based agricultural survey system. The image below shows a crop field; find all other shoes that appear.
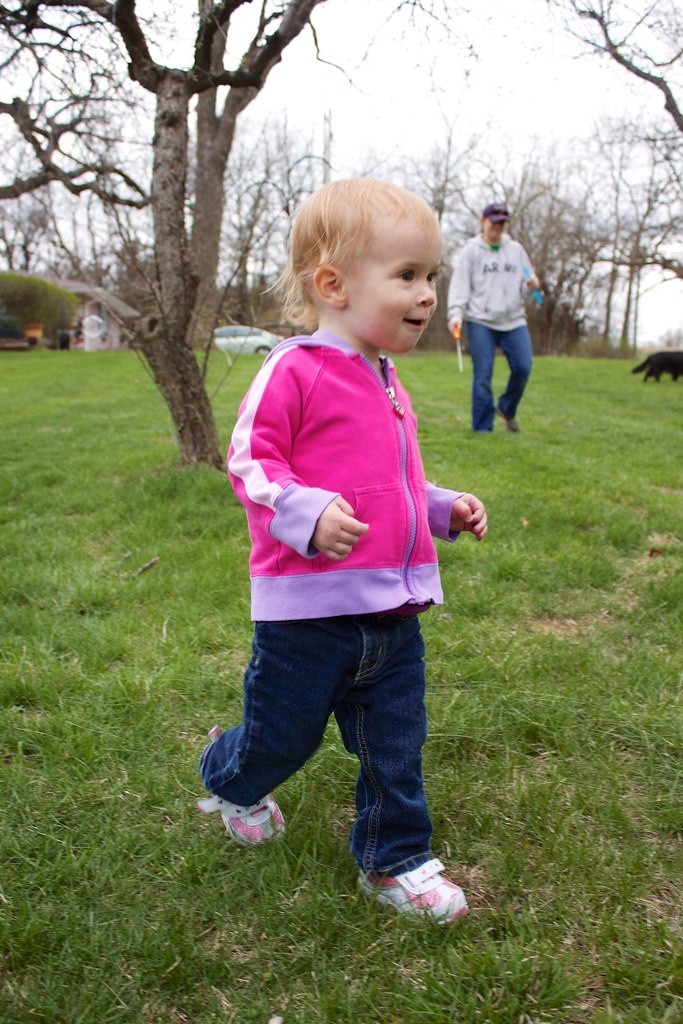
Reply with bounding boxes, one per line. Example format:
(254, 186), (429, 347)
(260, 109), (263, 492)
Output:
(495, 403), (519, 432)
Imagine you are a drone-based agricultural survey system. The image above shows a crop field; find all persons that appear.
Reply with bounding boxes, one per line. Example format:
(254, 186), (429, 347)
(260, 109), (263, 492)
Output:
(197, 180), (489, 925)
(447, 206), (540, 431)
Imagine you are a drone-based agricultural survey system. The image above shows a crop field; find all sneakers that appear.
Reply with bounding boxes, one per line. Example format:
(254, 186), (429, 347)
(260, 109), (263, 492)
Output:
(355, 858), (468, 927)
(197, 724), (286, 847)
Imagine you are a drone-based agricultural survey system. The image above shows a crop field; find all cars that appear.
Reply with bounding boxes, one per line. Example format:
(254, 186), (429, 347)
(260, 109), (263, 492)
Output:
(213, 324), (285, 356)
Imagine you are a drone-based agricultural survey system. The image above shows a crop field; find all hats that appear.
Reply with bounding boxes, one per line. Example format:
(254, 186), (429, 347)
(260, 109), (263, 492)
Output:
(484, 203), (510, 223)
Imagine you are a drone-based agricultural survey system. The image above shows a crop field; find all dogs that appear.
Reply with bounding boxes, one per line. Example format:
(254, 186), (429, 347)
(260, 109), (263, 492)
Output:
(632, 352), (683, 382)
